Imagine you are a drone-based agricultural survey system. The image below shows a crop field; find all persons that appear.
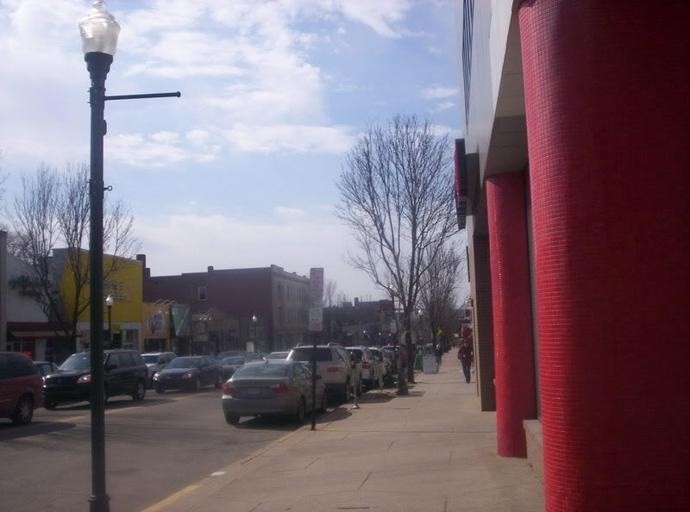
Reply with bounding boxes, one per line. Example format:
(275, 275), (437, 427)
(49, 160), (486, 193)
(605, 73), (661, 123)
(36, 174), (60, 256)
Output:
(458, 339), (474, 384)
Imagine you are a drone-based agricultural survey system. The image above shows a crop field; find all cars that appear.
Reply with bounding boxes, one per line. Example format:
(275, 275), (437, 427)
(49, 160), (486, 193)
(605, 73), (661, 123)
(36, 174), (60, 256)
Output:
(221, 359), (329, 424)
(32, 360), (59, 377)
(380, 344), (406, 373)
(141, 352), (178, 388)
(0, 350), (44, 428)
(219, 349), (288, 389)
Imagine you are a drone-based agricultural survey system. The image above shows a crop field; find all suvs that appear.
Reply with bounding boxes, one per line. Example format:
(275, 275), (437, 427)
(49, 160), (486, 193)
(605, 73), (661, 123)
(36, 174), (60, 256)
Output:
(347, 344), (392, 388)
(154, 355), (221, 394)
(42, 348), (150, 408)
(287, 340), (363, 405)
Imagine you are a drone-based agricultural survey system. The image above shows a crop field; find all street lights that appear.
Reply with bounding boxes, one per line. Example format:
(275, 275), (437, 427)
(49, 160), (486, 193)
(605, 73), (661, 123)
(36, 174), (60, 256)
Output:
(75, 1), (180, 512)
(252, 315), (259, 354)
(102, 291), (115, 349)
(417, 308), (425, 351)
